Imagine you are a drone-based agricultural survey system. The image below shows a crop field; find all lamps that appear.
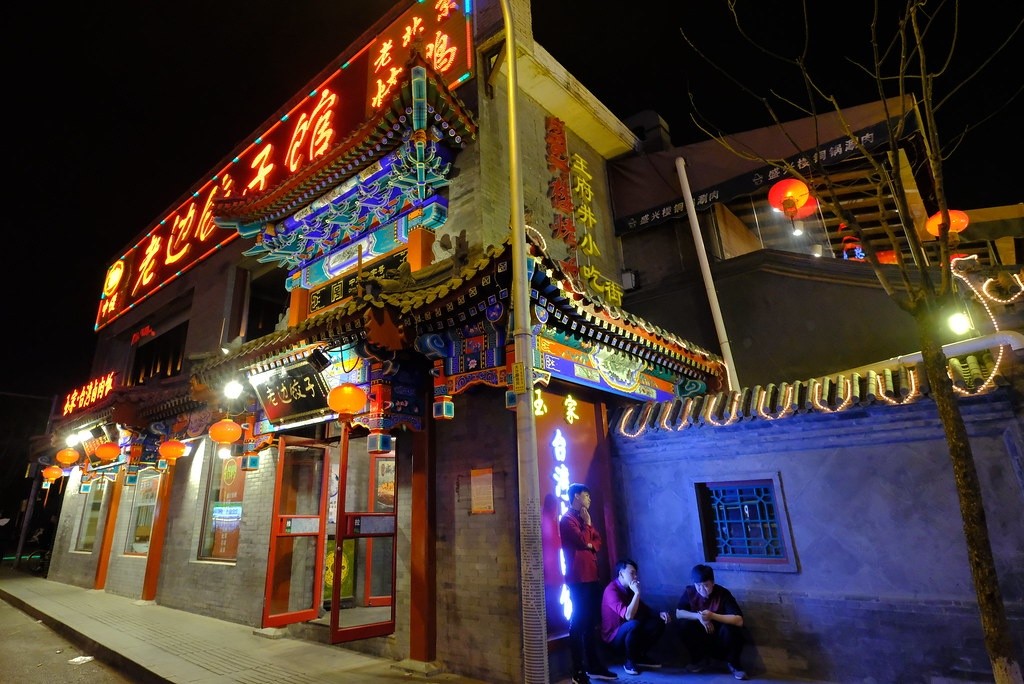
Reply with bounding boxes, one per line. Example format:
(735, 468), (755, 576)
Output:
(792, 221), (803, 235)
(811, 245), (823, 258)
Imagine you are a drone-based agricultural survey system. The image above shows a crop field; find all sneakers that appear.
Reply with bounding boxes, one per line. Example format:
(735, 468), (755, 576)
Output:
(728, 663), (748, 679)
(587, 670), (617, 679)
(634, 656), (661, 667)
(690, 663), (706, 672)
(624, 660), (638, 674)
(572, 674), (592, 684)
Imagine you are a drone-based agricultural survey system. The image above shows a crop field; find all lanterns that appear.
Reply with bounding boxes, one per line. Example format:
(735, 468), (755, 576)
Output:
(159, 439), (186, 466)
(925, 210), (969, 248)
(768, 179), (810, 218)
(209, 420), (243, 450)
(327, 383), (367, 424)
(43, 465), (63, 486)
(95, 443), (121, 465)
(56, 449), (80, 467)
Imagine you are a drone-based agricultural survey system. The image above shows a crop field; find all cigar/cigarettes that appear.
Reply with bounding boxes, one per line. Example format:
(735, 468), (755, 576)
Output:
(636, 580), (639, 584)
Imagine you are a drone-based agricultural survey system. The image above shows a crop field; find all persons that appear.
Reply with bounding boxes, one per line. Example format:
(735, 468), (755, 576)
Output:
(674, 565), (748, 680)
(558, 483), (671, 684)
